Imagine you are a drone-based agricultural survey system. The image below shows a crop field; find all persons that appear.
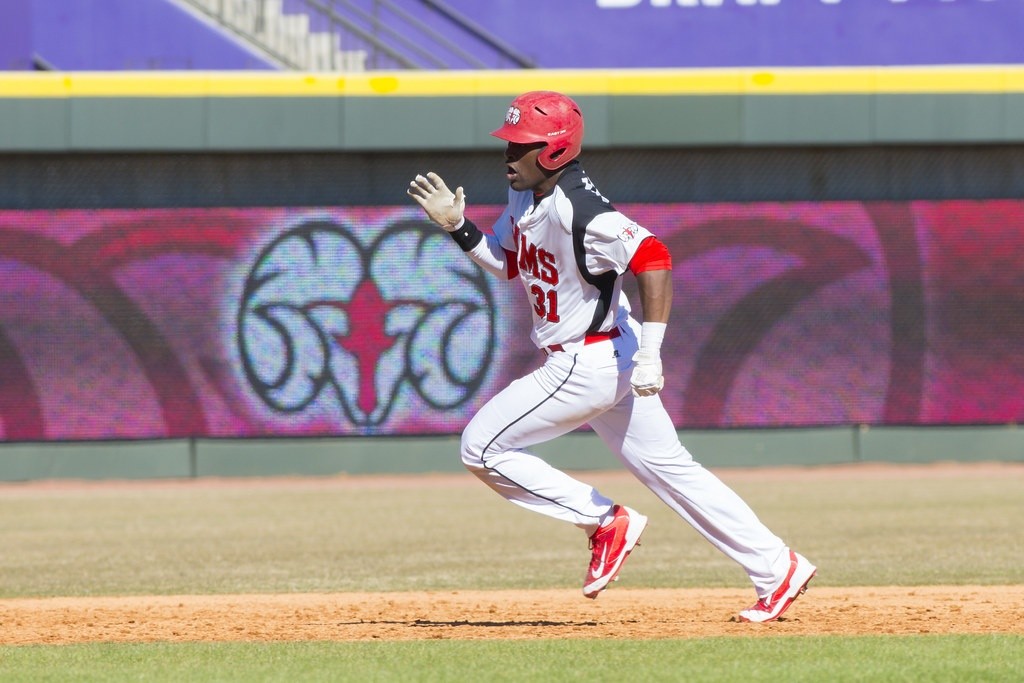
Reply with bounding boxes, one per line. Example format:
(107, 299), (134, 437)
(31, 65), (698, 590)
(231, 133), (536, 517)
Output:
(406, 91), (816, 621)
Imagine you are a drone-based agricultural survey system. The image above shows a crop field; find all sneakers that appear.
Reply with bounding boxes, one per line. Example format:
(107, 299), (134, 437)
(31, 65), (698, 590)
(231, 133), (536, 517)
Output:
(737, 546), (818, 624)
(582, 503), (650, 599)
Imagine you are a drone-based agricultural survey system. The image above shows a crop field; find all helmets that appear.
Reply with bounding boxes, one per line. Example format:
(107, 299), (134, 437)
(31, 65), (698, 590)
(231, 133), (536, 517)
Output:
(490, 90), (584, 171)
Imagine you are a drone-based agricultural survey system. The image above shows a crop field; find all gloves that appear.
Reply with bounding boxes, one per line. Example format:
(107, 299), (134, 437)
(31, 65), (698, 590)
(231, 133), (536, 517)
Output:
(628, 322), (664, 399)
(406, 171), (465, 236)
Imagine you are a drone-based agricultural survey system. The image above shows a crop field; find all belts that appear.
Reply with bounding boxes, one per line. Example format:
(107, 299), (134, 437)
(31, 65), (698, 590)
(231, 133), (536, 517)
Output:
(542, 325), (624, 357)
